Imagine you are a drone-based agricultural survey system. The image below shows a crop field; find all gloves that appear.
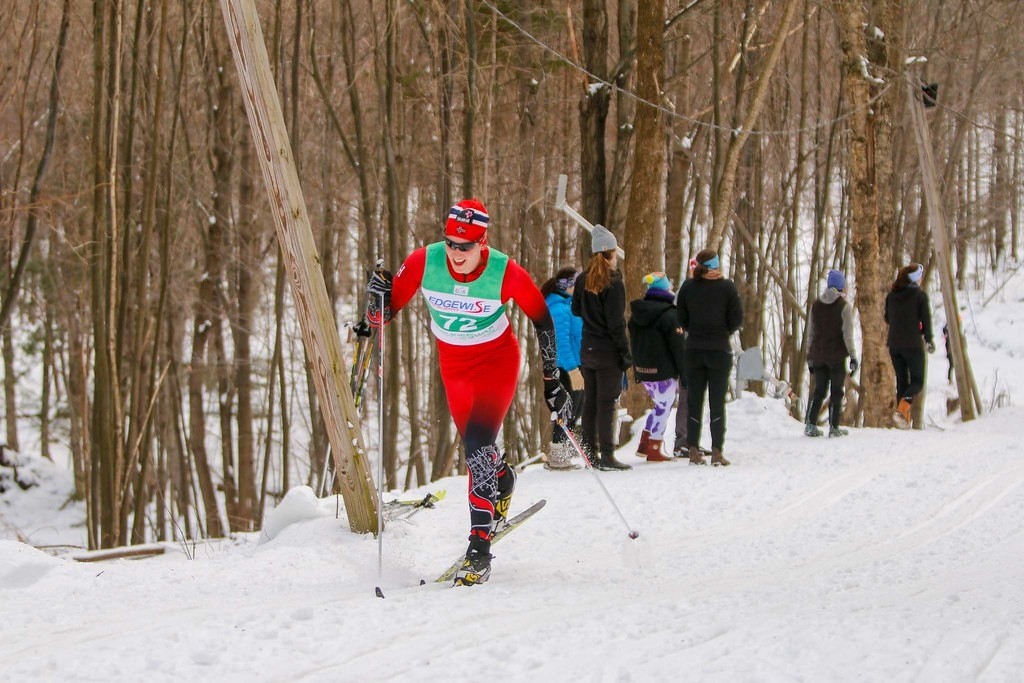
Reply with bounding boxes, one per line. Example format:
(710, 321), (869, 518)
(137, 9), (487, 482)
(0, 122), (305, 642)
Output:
(807, 360), (815, 374)
(543, 378), (573, 426)
(849, 359), (858, 377)
(368, 270), (392, 307)
(927, 340), (936, 354)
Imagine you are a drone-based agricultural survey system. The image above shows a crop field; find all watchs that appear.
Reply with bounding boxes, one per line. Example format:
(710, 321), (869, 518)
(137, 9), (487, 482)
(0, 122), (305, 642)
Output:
(542, 368), (561, 378)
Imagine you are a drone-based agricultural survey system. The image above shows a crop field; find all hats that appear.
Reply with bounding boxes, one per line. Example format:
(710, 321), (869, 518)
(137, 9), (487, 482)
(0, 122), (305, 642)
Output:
(642, 271), (671, 292)
(827, 269), (846, 290)
(591, 225), (617, 253)
(444, 197), (490, 242)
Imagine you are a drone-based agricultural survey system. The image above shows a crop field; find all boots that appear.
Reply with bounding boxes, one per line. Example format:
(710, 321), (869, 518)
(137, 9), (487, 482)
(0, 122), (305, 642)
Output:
(646, 437), (678, 462)
(542, 442), (582, 470)
(601, 450), (632, 471)
(584, 446), (608, 471)
(893, 398), (912, 430)
(689, 445), (706, 467)
(711, 447), (729, 466)
(636, 428), (651, 457)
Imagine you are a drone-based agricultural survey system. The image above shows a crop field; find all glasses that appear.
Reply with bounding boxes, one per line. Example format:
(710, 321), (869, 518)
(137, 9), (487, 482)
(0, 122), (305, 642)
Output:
(442, 234), (479, 251)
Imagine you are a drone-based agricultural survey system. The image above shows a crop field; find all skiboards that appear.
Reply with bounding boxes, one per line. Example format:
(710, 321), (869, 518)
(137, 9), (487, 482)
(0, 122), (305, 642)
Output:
(373, 498), (551, 601)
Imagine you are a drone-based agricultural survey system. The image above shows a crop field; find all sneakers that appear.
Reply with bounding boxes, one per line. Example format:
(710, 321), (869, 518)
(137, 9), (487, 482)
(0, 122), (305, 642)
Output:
(803, 423), (824, 437)
(453, 536), (490, 585)
(829, 425), (849, 438)
(492, 461), (517, 524)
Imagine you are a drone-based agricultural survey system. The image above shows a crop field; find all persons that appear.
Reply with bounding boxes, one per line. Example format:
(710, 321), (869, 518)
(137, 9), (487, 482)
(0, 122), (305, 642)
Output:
(884, 263), (936, 430)
(804, 268), (858, 438)
(677, 248), (743, 466)
(673, 333), (712, 458)
(627, 271), (680, 462)
(542, 265), (585, 471)
(571, 224), (633, 471)
(364, 197), (570, 587)
(943, 322), (954, 384)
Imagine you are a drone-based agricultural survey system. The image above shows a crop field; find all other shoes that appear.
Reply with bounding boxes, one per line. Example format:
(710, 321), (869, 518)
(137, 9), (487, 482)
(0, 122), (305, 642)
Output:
(675, 443), (712, 458)
